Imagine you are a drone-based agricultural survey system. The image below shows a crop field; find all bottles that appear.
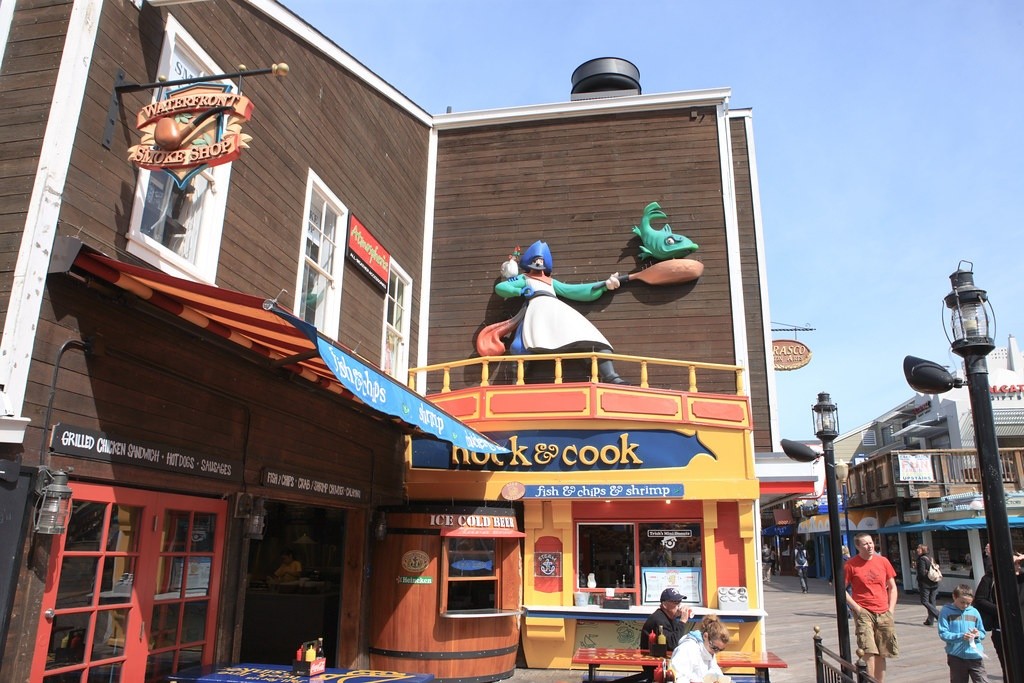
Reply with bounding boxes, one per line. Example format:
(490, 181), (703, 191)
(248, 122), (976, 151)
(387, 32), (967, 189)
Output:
(589, 594), (632, 605)
(587, 573), (597, 589)
(649, 630), (667, 645)
(296, 637), (324, 662)
(61, 632), (81, 649)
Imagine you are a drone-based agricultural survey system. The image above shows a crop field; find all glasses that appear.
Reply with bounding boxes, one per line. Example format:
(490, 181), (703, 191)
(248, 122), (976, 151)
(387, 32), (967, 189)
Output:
(707, 636), (723, 652)
(672, 600), (681, 604)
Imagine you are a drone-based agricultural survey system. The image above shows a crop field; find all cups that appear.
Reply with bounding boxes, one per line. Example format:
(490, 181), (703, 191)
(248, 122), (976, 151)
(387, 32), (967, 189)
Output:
(606, 588), (614, 596)
(678, 605), (695, 619)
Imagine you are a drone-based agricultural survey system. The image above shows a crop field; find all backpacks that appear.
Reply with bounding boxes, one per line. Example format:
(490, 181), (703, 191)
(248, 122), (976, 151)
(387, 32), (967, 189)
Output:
(796, 548), (806, 565)
(922, 555), (942, 582)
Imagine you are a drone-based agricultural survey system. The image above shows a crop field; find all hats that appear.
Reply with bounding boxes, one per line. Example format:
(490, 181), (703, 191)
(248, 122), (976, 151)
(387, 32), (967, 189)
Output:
(796, 541), (804, 547)
(660, 588), (687, 602)
(1012, 552), (1024, 563)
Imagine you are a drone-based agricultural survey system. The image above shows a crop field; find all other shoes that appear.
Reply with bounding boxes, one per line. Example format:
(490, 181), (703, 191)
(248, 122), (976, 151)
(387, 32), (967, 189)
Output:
(802, 590), (807, 593)
(847, 612), (852, 619)
(765, 579), (771, 583)
(763, 578), (767, 582)
(924, 619), (932, 625)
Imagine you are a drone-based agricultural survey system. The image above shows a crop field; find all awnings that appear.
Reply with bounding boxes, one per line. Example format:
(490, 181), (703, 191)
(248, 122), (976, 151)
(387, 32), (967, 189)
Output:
(797, 509), (880, 534)
(761, 525), (793, 535)
(71, 251), (512, 456)
(877, 515), (1024, 534)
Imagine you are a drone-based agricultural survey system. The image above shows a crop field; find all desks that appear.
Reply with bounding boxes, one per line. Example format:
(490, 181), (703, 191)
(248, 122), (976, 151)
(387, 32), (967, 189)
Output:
(167, 663), (434, 683)
(572, 648), (787, 683)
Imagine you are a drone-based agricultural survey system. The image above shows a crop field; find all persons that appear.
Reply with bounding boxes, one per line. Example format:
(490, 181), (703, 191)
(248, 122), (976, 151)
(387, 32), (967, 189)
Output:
(971, 543), (1024, 683)
(828, 533), (897, 683)
(916, 544), (942, 625)
(666, 613), (730, 683)
(640, 539), (672, 566)
(274, 547), (302, 593)
(938, 584), (992, 683)
(641, 588), (689, 679)
(792, 542), (810, 594)
(761, 544), (777, 576)
(494, 241), (620, 385)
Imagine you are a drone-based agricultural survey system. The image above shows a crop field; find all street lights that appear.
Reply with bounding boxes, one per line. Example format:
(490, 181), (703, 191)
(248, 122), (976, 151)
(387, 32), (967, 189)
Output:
(904, 267), (1024, 683)
(780, 390), (858, 676)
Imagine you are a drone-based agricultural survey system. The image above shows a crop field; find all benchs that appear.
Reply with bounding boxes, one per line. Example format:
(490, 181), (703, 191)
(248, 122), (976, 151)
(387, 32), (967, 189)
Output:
(580, 671), (770, 683)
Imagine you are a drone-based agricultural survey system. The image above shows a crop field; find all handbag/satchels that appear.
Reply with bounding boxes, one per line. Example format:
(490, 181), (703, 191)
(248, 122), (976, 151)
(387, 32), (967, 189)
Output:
(971, 600), (998, 631)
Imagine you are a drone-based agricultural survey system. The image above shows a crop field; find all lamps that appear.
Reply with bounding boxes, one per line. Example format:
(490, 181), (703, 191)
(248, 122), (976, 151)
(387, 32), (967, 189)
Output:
(242, 498), (266, 539)
(374, 512), (387, 544)
(292, 533), (318, 545)
(30, 465), (72, 535)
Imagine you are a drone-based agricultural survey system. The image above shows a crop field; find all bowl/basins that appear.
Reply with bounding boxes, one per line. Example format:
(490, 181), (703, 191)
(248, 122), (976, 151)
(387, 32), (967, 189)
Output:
(575, 592), (590, 606)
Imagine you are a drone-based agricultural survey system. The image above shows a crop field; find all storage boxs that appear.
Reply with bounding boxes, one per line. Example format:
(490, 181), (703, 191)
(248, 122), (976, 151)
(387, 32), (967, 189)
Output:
(603, 596), (631, 609)
